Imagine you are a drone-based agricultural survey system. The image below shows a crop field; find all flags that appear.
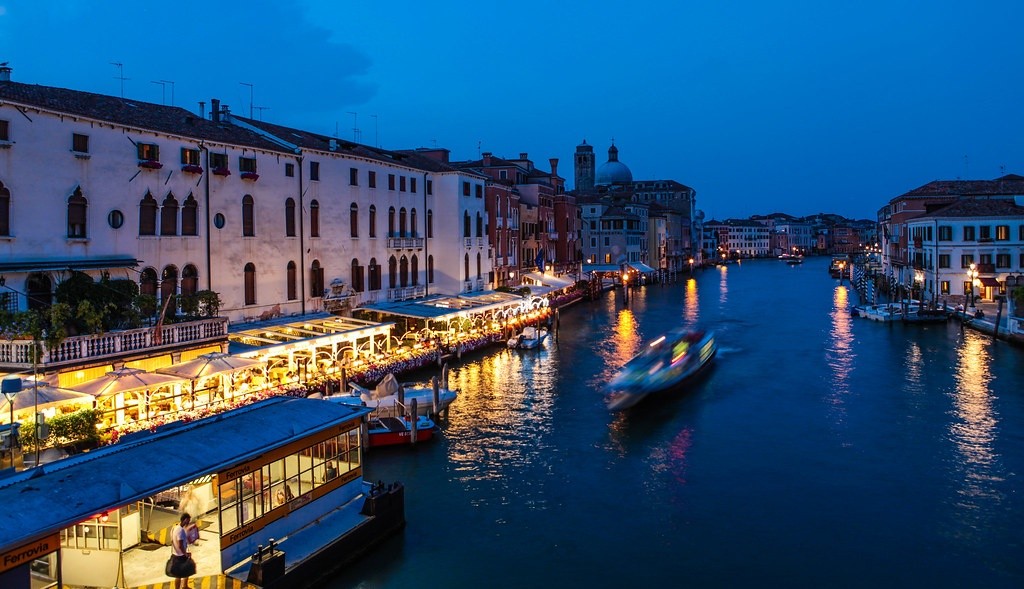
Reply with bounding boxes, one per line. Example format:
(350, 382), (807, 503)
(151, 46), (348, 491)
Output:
(155, 298), (170, 343)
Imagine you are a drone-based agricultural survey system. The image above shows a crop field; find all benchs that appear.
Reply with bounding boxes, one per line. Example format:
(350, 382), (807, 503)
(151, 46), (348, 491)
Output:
(212, 468), (271, 506)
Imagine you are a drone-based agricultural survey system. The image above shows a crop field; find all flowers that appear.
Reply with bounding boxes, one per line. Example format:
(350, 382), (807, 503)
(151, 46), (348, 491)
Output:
(138, 160), (260, 181)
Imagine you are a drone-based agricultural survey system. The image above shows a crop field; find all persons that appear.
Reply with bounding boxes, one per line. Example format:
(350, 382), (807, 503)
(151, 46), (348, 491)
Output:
(323, 461), (336, 481)
(974, 309), (984, 319)
(958, 301), (962, 309)
(276, 485), (294, 504)
(171, 513), (192, 589)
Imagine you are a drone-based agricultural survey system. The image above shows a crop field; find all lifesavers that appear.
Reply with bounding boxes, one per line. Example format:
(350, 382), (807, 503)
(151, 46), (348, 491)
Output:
(405, 416), (419, 421)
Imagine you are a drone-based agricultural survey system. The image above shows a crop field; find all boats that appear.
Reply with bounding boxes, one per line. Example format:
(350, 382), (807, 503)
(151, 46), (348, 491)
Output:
(507, 327), (548, 350)
(307, 363), (458, 449)
(607, 322), (718, 413)
(785, 256), (803, 264)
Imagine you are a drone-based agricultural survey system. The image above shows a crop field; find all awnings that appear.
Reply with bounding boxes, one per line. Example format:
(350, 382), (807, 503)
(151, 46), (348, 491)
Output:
(629, 263), (655, 272)
(582, 265), (620, 271)
(514, 272), (592, 297)
(980, 277), (1002, 286)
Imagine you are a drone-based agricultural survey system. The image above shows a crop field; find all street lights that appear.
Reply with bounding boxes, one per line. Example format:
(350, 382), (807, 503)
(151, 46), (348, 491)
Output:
(967, 263), (978, 308)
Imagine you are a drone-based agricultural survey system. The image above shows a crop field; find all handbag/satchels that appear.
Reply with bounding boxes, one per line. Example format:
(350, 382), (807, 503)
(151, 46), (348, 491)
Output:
(165, 557), (195, 578)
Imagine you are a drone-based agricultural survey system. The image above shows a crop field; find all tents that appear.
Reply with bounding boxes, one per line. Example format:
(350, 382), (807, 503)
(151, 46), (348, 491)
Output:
(0, 351), (259, 416)
